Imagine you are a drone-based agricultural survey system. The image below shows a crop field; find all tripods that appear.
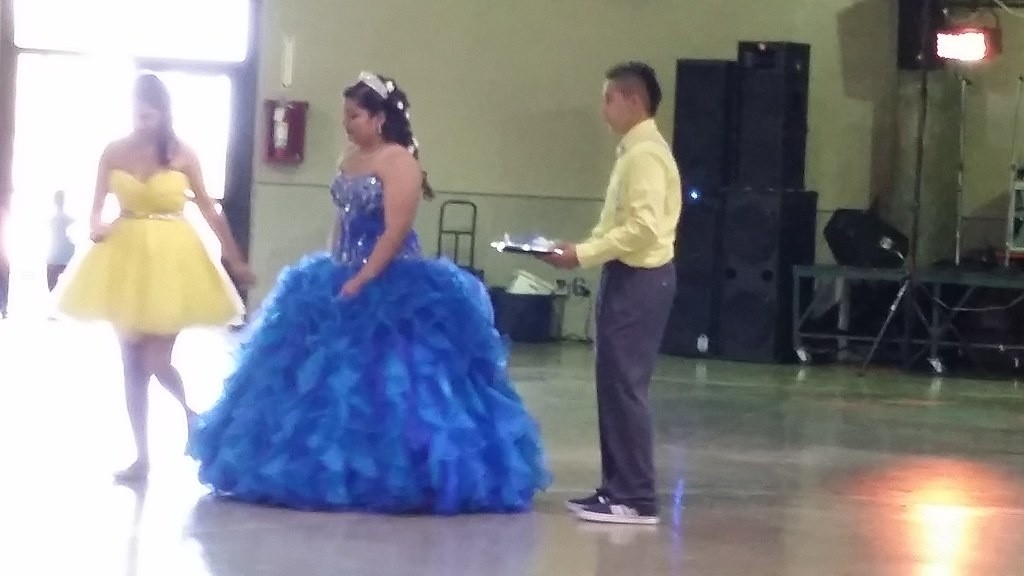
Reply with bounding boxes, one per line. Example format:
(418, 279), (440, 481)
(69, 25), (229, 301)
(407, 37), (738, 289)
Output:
(861, 71), (988, 378)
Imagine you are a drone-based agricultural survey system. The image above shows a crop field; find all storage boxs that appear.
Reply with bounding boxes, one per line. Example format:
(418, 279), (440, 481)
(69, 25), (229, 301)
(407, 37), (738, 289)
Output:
(508, 269), (554, 295)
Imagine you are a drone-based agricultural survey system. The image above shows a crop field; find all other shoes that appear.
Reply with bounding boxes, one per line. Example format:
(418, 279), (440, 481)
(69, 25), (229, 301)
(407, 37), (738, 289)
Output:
(112, 459), (150, 483)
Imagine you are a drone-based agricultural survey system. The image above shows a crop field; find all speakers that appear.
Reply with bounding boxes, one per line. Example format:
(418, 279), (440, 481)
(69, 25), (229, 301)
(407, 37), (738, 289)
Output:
(670, 41), (819, 360)
(895, 0), (944, 72)
(824, 210), (911, 271)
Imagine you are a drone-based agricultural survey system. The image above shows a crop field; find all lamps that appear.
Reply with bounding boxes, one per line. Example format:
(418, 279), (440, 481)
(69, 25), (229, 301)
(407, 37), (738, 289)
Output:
(935, 9), (1002, 61)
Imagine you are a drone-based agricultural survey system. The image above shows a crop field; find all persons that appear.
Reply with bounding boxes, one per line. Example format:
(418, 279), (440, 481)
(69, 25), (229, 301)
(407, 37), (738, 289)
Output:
(329, 70), (423, 512)
(60, 76), (259, 478)
(46, 191), (75, 291)
(539, 62), (681, 524)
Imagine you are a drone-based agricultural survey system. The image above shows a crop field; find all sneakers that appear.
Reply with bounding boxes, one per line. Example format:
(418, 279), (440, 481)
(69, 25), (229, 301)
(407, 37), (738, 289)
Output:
(566, 489), (663, 526)
